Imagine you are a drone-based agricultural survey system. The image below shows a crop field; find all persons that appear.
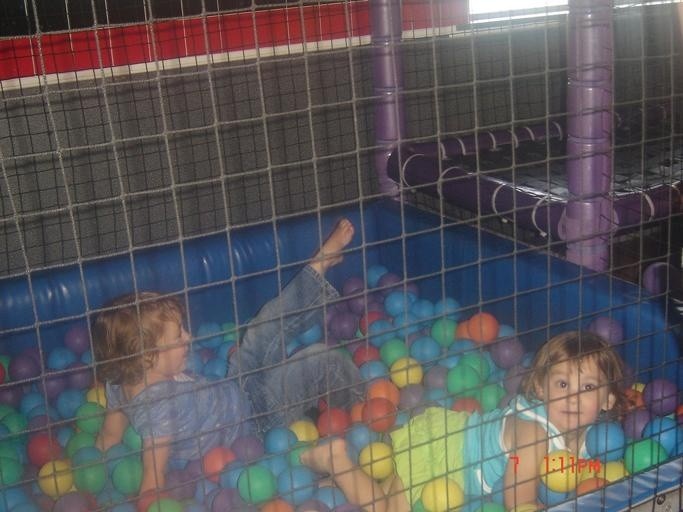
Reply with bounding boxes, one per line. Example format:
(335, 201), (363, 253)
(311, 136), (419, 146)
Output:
(95, 192), (374, 511)
